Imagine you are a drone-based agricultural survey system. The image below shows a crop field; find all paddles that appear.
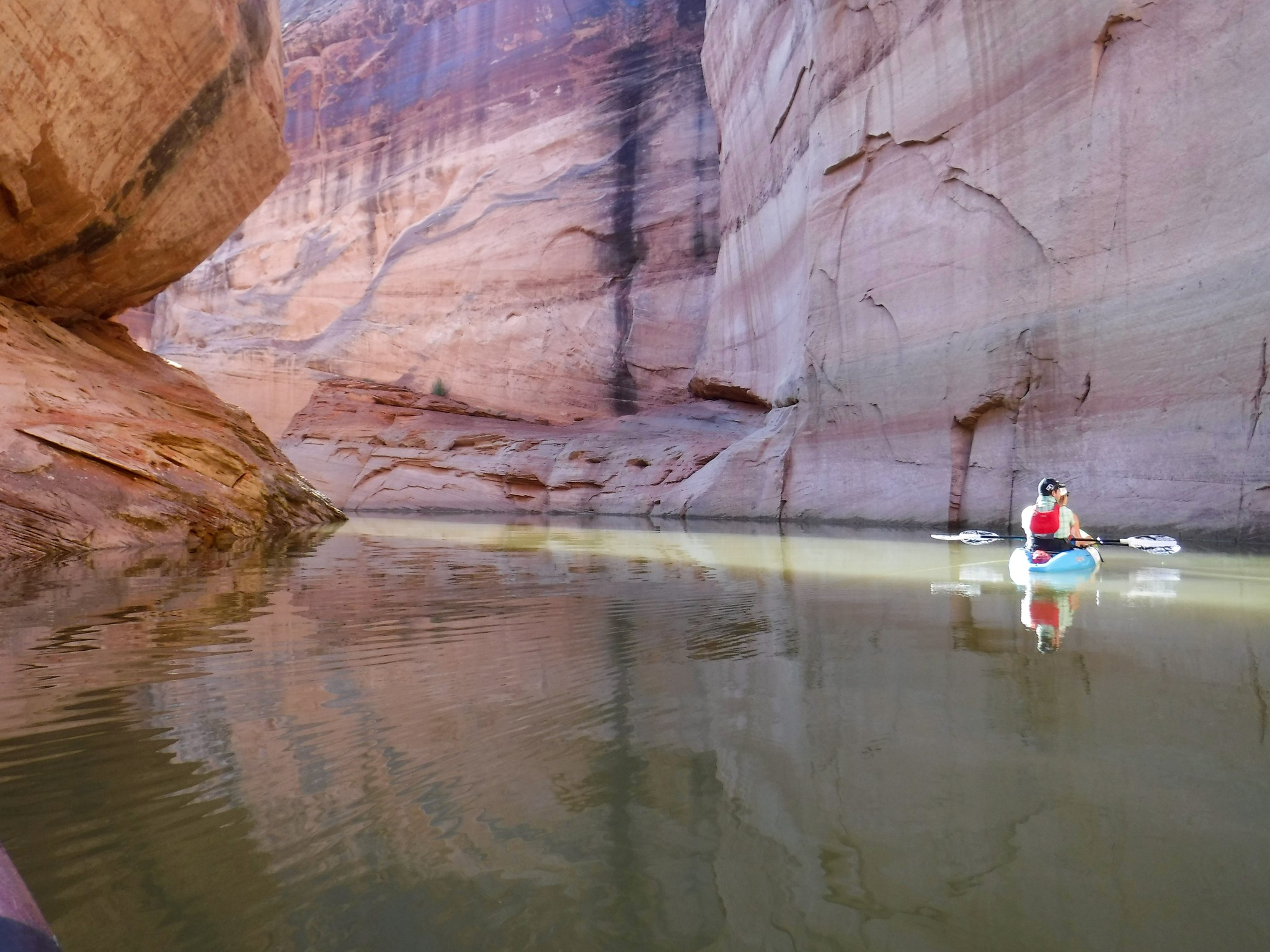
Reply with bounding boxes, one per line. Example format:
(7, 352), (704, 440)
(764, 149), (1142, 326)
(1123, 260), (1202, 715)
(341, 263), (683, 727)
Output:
(930, 533), (1178, 549)
(959, 529), (1182, 554)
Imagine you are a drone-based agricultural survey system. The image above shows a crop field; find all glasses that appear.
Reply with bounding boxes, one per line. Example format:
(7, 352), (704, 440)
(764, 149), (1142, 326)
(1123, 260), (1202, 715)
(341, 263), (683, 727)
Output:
(1061, 491), (1070, 497)
(1050, 480), (1059, 490)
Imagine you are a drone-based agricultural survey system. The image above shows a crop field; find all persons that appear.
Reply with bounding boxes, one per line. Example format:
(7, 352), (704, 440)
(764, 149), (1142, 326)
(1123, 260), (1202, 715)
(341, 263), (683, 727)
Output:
(1021, 477), (1097, 551)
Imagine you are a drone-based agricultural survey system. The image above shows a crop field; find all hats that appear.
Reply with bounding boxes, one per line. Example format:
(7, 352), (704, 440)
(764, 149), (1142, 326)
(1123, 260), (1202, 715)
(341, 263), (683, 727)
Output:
(1038, 478), (1066, 496)
(1036, 636), (1058, 654)
(1059, 484), (1070, 496)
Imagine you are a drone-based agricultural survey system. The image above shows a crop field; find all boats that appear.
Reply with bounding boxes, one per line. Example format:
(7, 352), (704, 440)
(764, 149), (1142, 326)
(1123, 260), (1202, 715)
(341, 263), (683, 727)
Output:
(1011, 577), (1100, 594)
(1008, 528), (1105, 576)
